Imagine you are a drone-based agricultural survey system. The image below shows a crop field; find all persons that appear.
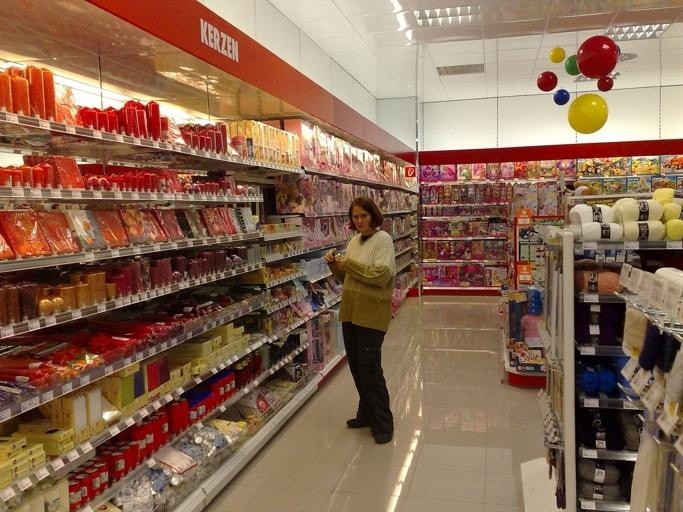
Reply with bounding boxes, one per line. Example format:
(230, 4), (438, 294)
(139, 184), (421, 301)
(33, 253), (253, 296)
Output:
(323, 195), (395, 444)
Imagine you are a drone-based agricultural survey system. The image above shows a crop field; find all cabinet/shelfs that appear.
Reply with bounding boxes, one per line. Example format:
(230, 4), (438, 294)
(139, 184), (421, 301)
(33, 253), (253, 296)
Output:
(498, 173), (682, 510)
(0, 112), (417, 512)
(420, 169), (511, 297)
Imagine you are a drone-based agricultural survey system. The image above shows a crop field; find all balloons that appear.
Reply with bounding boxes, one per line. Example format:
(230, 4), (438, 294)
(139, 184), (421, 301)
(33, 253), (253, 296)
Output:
(535, 35), (621, 134)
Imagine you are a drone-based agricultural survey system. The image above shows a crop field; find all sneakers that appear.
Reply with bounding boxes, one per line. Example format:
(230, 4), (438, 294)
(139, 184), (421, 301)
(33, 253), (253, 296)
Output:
(374, 427), (393, 443)
(347, 419), (369, 429)
